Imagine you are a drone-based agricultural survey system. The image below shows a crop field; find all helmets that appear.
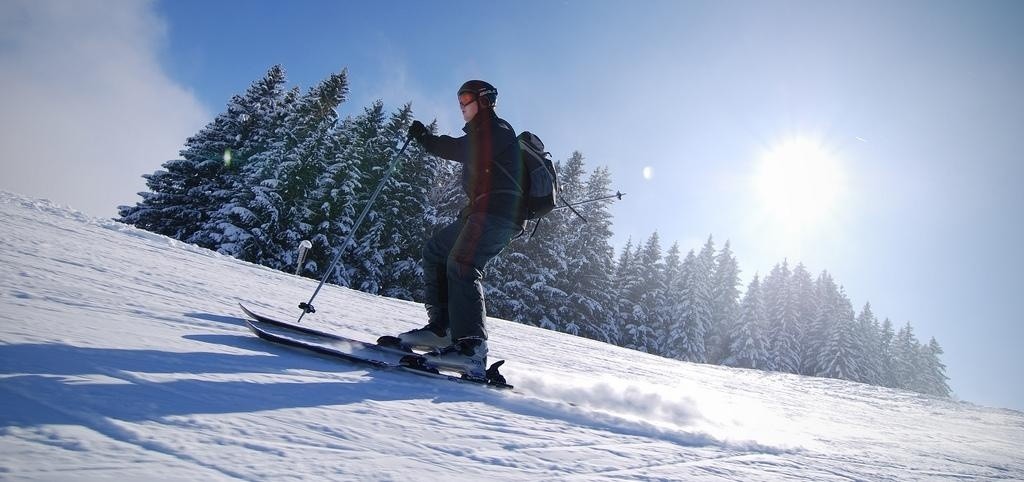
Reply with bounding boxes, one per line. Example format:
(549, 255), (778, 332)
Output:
(457, 80), (498, 108)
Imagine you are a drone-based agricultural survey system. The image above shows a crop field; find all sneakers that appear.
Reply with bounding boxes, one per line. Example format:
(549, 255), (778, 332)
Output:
(423, 337), (489, 379)
(399, 325), (454, 350)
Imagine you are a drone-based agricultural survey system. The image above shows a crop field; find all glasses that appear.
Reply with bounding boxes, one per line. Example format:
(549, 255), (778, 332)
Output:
(458, 92), (477, 106)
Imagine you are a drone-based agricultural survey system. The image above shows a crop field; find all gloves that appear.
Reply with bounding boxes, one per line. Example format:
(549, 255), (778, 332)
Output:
(408, 120), (427, 142)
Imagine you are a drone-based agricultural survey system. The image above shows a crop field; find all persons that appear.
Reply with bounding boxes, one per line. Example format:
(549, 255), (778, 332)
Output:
(396, 79), (529, 374)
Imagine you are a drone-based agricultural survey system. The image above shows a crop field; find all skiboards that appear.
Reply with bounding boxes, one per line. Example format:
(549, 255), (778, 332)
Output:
(237, 303), (524, 396)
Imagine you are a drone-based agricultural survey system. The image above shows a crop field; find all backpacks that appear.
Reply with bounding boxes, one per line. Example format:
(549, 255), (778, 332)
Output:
(493, 122), (559, 218)
